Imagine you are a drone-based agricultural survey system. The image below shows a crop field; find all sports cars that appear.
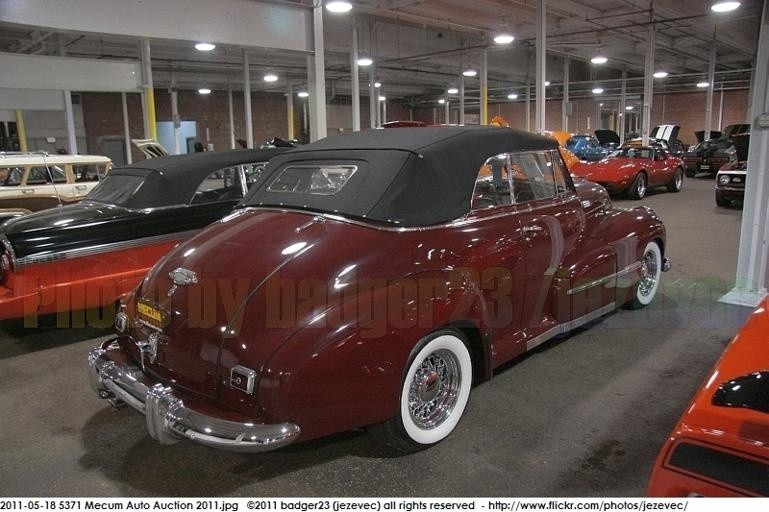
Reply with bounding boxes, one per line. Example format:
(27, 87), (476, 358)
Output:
(569, 144), (685, 201)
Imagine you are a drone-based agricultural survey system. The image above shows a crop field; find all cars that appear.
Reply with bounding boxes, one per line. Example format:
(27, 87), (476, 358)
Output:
(645, 293), (769, 497)
(0, 138), (169, 227)
(380, 116), (690, 183)
(681, 124), (750, 179)
(715, 133), (751, 209)
(0, 147), (298, 321)
(252, 138), (309, 189)
(87, 126), (671, 455)
